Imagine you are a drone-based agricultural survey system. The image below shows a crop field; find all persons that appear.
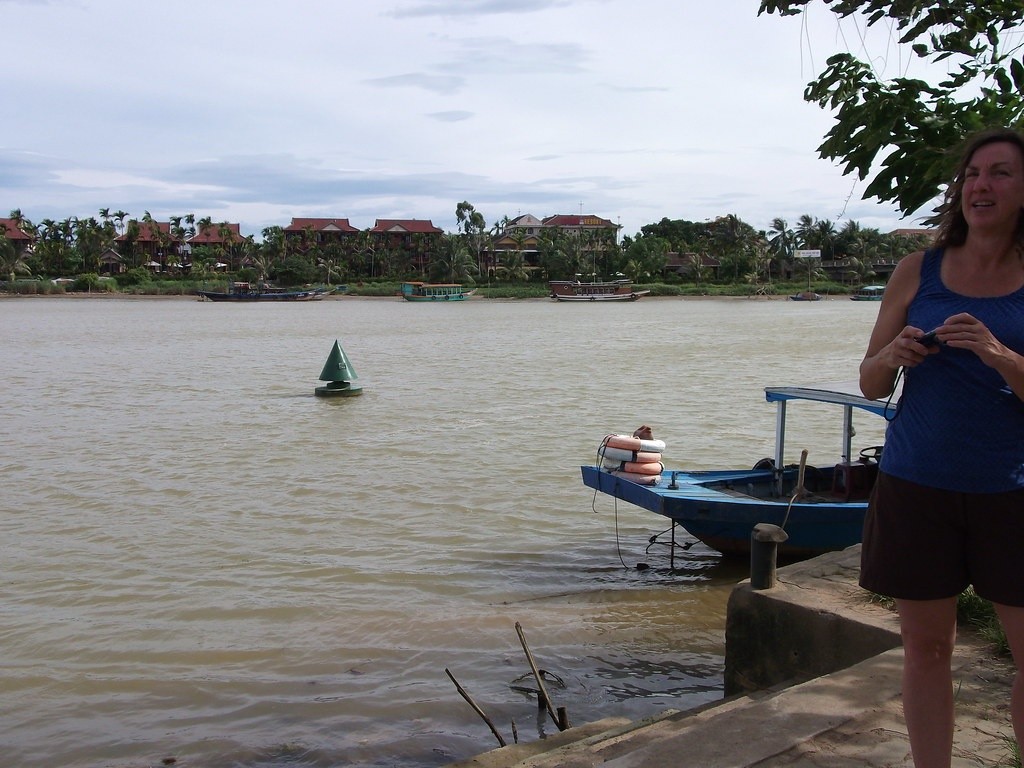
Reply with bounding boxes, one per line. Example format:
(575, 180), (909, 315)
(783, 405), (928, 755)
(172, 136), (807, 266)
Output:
(859, 129), (1023, 768)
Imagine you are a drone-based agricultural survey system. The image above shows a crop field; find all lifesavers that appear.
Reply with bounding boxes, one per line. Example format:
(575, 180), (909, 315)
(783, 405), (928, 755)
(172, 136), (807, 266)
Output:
(599, 434), (666, 484)
(459, 295), (463, 299)
(255, 293), (260, 297)
(432, 296), (436, 300)
(272, 295), (278, 300)
(631, 294), (636, 299)
(564, 286), (568, 289)
(445, 296), (449, 300)
(201, 293), (204, 298)
(590, 297), (595, 302)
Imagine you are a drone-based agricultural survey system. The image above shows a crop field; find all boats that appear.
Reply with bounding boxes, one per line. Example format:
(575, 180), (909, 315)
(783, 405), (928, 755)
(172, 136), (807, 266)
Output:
(580, 384), (905, 570)
(549, 278), (635, 299)
(849, 286), (887, 302)
(550, 290), (651, 303)
(399, 281), (479, 302)
(790, 292), (822, 301)
(197, 281), (338, 301)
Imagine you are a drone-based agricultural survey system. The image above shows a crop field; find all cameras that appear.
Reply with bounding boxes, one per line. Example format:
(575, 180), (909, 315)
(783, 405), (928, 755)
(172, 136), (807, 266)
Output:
(914, 328), (942, 348)
